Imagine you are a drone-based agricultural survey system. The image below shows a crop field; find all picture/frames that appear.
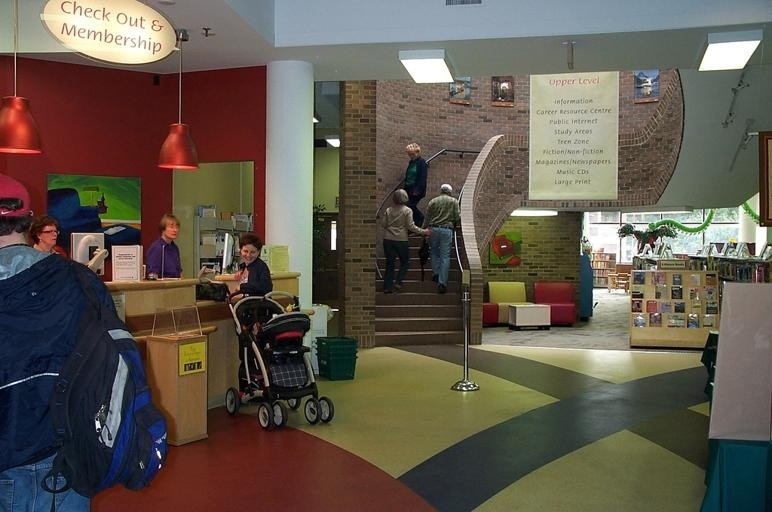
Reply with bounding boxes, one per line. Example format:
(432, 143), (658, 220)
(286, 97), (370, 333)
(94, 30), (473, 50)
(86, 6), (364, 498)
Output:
(449, 76), (471, 104)
(492, 76), (514, 107)
(634, 69), (660, 103)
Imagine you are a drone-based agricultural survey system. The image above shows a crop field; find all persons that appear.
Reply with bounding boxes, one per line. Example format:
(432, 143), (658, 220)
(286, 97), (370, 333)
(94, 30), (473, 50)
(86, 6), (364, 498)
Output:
(235, 234), (272, 299)
(422, 183), (459, 296)
(146, 213), (183, 277)
(0, 173), (118, 512)
(29, 216), (68, 260)
(382, 189), (432, 294)
(404, 143), (428, 237)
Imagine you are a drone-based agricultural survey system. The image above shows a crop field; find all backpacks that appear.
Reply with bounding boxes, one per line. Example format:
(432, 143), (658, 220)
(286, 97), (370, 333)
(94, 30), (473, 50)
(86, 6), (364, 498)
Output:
(40, 260), (168, 512)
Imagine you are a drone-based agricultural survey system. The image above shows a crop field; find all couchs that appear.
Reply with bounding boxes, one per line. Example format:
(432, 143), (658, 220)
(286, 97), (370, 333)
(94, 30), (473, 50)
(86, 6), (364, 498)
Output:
(534, 280), (576, 327)
(488, 281), (535, 327)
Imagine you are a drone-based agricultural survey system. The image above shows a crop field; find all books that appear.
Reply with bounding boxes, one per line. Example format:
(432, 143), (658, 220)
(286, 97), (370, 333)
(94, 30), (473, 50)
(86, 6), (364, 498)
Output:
(632, 256), (768, 328)
(590, 254), (611, 287)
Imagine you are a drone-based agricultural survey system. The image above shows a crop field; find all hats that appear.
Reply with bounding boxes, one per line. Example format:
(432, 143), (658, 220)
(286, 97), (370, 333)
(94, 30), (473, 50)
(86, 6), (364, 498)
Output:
(0, 174), (32, 217)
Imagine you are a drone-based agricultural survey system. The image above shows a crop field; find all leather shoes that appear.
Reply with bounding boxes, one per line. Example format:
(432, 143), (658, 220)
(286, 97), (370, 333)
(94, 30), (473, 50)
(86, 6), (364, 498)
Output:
(384, 289), (394, 293)
(394, 283), (402, 289)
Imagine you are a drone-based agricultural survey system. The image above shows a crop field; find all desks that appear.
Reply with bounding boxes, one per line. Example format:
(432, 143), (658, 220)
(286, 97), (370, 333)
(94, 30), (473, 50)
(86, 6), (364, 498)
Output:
(608, 274), (631, 294)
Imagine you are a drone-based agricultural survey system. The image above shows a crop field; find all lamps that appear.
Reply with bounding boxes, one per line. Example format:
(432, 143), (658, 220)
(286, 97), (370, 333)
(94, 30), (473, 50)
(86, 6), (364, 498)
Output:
(159, 34), (199, 169)
(398, 49), (456, 83)
(1, 0), (43, 153)
(696, 29), (764, 72)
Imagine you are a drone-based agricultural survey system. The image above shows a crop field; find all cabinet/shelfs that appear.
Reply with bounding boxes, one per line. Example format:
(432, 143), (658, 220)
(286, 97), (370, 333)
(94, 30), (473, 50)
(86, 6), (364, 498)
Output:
(709, 256), (772, 297)
(628, 270), (719, 351)
(632, 256), (689, 270)
(688, 255), (707, 271)
(194, 216), (252, 280)
(592, 252), (616, 288)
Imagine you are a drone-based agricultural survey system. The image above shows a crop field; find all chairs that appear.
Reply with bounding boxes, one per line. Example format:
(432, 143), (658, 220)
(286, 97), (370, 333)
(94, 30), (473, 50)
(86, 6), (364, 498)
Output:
(615, 273), (628, 292)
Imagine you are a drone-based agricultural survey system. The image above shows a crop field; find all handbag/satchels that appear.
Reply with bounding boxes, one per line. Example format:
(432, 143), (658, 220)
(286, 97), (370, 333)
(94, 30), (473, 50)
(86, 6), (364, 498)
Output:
(196, 281), (231, 302)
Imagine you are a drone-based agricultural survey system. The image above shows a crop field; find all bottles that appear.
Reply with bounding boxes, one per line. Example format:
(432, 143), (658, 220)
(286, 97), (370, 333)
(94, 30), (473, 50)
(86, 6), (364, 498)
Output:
(235, 261), (239, 271)
(213, 262), (220, 275)
(227, 264), (230, 273)
(715, 259), (765, 282)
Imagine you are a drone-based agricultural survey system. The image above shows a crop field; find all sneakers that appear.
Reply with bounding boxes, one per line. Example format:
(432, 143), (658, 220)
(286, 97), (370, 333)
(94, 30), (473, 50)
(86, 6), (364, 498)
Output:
(438, 284), (447, 294)
(432, 274), (439, 283)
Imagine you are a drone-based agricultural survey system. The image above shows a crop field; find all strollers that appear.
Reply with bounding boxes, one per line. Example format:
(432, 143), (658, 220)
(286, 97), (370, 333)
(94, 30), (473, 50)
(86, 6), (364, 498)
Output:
(225, 289), (335, 431)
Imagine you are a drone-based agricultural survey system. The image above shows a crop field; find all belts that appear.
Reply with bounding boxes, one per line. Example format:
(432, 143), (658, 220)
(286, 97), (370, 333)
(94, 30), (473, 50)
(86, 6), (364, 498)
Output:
(429, 224), (452, 229)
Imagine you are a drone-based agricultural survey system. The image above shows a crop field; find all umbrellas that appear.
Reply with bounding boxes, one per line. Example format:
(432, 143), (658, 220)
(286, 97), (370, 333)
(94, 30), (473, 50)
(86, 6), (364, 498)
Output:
(418, 236), (429, 281)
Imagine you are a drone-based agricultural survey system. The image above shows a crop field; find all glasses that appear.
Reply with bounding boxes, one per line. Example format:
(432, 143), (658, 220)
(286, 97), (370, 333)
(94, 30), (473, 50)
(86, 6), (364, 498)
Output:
(40, 229), (60, 235)
